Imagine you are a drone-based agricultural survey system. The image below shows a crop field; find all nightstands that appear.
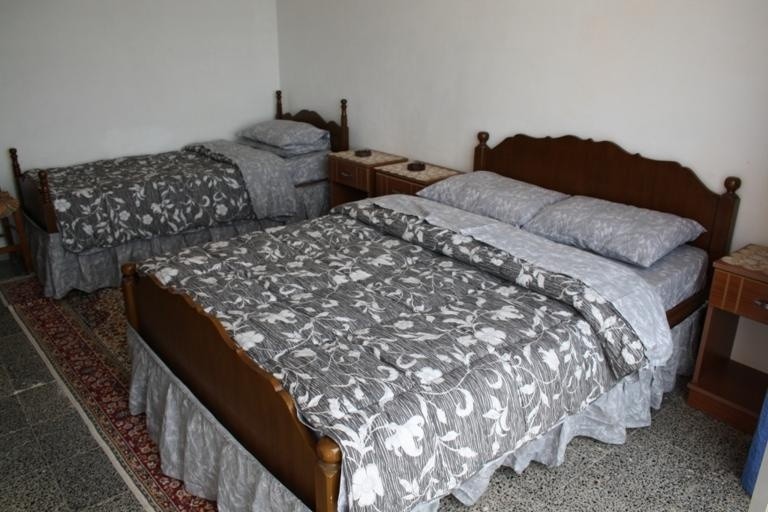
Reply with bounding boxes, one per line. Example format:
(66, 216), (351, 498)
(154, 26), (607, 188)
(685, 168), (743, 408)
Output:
(374, 158), (465, 197)
(685, 245), (768, 434)
(328, 148), (409, 214)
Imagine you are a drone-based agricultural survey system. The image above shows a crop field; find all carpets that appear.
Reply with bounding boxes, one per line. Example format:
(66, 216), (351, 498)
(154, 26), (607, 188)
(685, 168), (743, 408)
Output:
(0, 273), (219, 511)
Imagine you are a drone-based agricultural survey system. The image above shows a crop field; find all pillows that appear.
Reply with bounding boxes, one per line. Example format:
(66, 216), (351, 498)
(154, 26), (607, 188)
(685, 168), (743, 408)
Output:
(518, 194), (704, 268)
(237, 119), (330, 158)
(415, 169), (568, 226)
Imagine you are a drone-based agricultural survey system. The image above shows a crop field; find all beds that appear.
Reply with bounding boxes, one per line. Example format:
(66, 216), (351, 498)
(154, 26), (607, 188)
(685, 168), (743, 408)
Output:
(121, 132), (741, 510)
(9, 90), (352, 298)
